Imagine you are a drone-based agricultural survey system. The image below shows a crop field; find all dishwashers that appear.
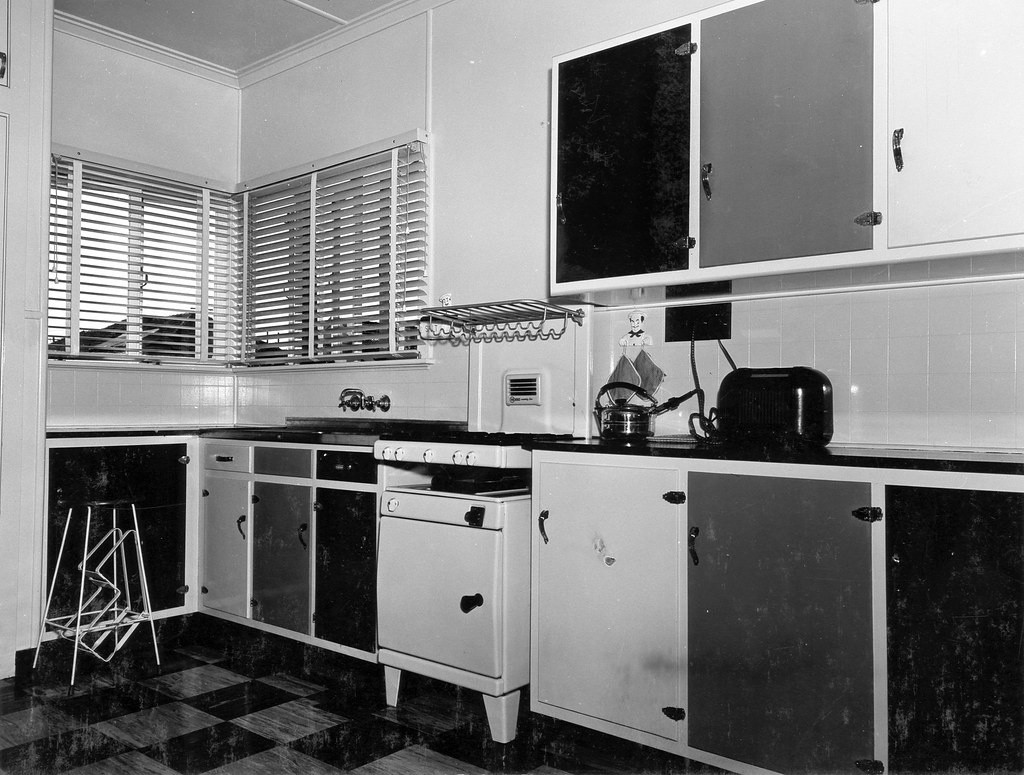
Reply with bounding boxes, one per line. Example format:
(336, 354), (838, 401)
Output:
(376, 484), (530, 744)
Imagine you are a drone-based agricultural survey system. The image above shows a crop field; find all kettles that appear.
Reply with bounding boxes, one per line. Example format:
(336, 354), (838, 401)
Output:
(595, 382), (659, 441)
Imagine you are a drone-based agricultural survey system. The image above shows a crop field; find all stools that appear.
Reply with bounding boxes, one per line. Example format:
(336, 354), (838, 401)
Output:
(31, 496), (163, 697)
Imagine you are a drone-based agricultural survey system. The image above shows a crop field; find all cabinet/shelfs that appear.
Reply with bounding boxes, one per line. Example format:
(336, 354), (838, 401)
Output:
(696, 0), (884, 282)
(198, 438), (252, 627)
(250, 441), (379, 665)
(884, 0), (1024, 264)
(551, 18), (698, 296)
(685, 458), (885, 775)
(883, 466), (1024, 775)
(529, 451), (687, 757)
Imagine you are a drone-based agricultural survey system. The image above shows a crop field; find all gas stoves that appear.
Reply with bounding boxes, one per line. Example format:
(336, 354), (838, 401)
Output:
(373, 430), (574, 469)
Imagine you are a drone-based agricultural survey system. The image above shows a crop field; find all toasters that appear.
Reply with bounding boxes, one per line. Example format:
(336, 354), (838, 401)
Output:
(717, 369), (834, 446)
